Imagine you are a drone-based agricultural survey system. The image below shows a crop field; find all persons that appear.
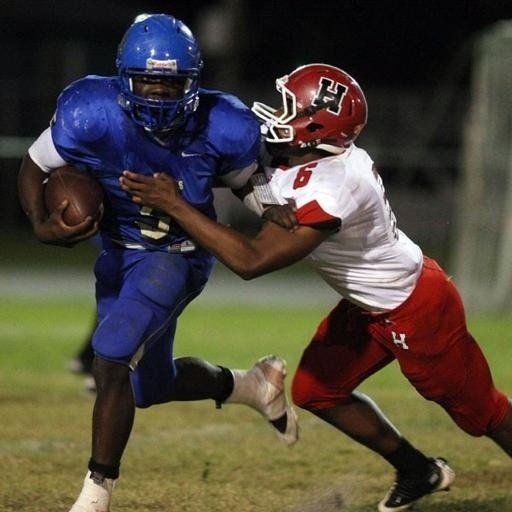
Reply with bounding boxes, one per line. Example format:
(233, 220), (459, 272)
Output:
(17, 10), (299, 512)
(119, 62), (512, 512)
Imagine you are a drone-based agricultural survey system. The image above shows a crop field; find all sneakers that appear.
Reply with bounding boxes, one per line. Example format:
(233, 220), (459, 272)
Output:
(251, 355), (300, 446)
(378, 457), (455, 512)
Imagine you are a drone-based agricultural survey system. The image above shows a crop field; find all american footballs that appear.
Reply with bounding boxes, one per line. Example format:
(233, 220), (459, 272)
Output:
(45, 168), (102, 237)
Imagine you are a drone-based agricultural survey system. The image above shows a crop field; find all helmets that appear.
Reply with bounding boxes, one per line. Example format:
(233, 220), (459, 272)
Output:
(252, 64), (368, 160)
(117, 14), (203, 127)
